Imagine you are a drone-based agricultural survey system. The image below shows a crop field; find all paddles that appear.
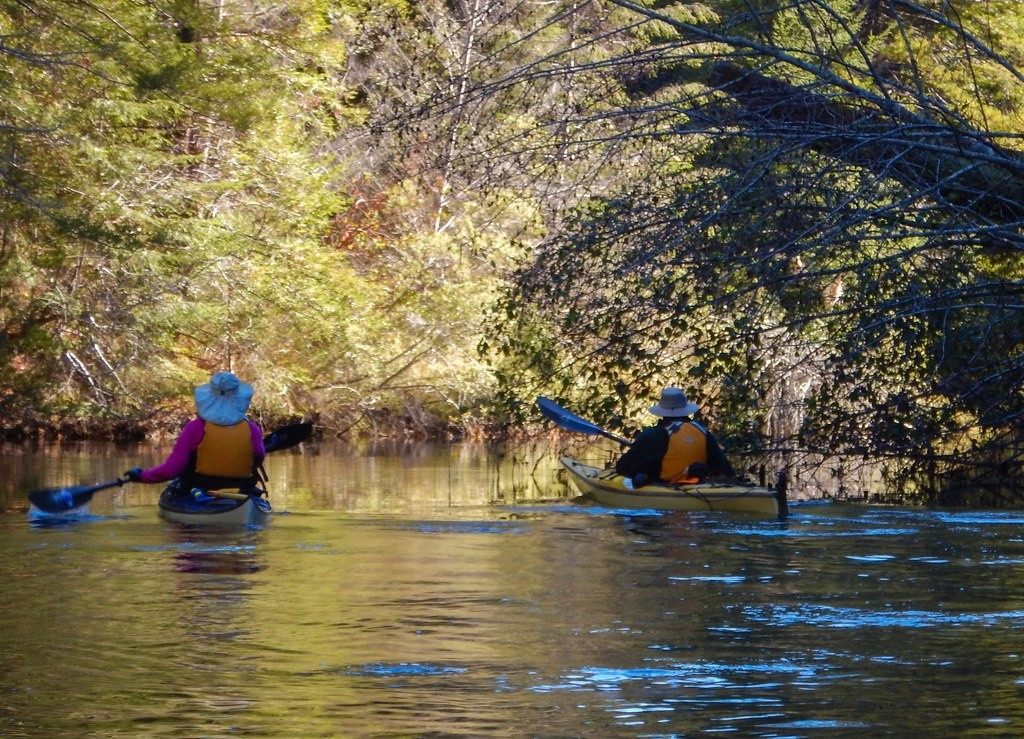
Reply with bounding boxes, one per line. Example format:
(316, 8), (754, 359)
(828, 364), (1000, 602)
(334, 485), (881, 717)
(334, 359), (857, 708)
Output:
(26, 471), (137, 514)
(541, 397), (631, 448)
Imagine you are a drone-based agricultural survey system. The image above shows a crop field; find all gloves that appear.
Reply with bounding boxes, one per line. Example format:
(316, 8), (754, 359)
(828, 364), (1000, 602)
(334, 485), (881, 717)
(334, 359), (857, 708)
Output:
(124, 466), (142, 482)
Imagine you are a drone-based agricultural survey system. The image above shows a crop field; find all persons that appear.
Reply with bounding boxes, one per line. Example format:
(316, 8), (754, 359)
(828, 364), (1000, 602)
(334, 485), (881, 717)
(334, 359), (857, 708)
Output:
(617, 387), (735, 482)
(123, 372), (266, 489)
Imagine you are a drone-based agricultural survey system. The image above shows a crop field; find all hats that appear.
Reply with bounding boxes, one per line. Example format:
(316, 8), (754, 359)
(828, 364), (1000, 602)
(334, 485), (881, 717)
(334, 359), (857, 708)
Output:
(648, 388), (699, 417)
(194, 372), (253, 426)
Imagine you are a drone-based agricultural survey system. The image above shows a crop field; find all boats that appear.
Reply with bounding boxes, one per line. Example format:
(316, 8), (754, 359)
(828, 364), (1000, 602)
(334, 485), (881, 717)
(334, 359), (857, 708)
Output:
(157, 480), (274, 534)
(559, 457), (786, 528)
